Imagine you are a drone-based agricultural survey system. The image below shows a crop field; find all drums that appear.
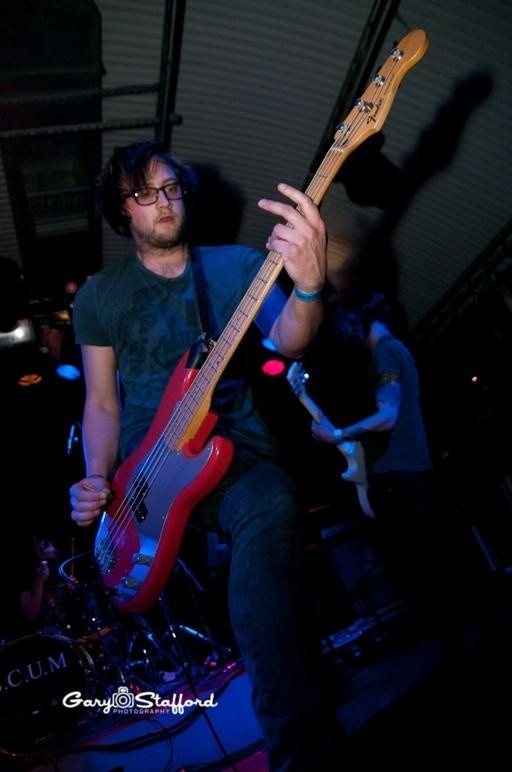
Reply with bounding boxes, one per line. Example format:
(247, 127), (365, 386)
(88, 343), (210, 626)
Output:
(0, 634), (97, 758)
(78, 628), (121, 675)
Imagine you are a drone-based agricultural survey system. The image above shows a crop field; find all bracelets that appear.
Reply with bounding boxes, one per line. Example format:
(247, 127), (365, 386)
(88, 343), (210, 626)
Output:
(333, 428), (343, 443)
(294, 284), (326, 301)
(85, 474), (104, 479)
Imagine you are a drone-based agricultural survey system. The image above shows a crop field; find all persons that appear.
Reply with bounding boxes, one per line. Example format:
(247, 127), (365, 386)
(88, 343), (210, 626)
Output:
(67, 138), (330, 771)
(12, 535), (81, 635)
(0, 256), (29, 334)
(308, 319), (434, 597)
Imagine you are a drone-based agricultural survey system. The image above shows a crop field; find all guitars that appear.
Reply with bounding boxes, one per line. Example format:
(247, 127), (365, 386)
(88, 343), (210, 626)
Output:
(287, 360), (382, 515)
(93, 28), (429, 611)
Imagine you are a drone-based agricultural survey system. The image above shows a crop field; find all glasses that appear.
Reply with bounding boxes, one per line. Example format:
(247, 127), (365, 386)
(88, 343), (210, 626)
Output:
(123, 182), (185, 206)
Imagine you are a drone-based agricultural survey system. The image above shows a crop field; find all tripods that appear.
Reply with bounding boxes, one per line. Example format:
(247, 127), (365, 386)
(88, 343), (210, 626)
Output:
(108, 622), (236, 689)
